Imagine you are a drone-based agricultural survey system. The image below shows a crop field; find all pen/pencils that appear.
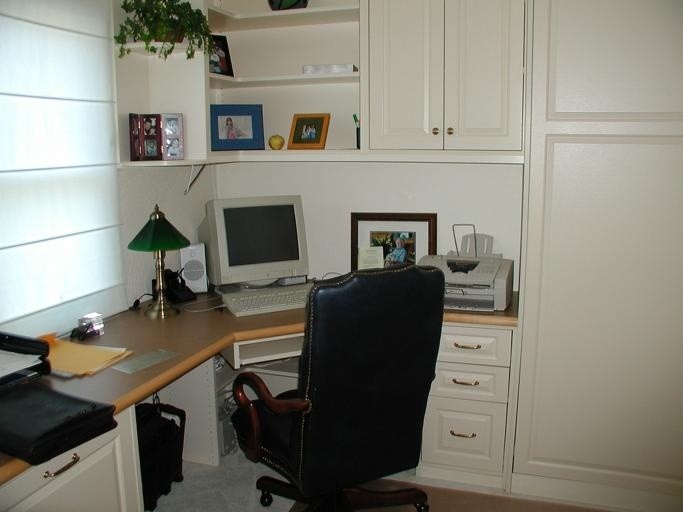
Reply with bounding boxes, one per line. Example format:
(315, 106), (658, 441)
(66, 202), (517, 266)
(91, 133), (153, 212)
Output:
(353, 114), (360, 129)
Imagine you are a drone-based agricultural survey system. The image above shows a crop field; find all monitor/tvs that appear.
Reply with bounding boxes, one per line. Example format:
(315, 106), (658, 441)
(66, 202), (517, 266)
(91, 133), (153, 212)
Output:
(196, 195), (309, 296)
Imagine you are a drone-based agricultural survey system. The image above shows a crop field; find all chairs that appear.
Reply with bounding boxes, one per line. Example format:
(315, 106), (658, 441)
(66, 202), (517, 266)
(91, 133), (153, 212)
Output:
(227, 265), (447, 510)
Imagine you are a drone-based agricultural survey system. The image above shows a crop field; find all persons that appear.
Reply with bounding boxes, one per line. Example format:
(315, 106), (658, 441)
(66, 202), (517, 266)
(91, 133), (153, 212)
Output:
(216, 46), (226, 69)
(301, 123), (317, 140)
(144, 119), (156, 136)
(166, 120), (176, 132)
(166, 140), (179, 156)
(384, 238), (407, 267)
(146, 140), (156, 156)
(225, 117), (247, 139)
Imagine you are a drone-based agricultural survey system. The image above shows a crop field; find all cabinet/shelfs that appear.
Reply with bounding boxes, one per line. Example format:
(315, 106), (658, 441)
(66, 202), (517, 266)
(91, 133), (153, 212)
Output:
(417, 307), (519, 489)
(208, 0), (363, 164)
(361, 1), (526, 164)
(0, 405), (146, 510)
(110, 0), (209, 169)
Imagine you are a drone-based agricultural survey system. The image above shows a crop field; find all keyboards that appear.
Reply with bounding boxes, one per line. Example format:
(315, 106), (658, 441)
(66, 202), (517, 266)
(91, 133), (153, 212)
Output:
(221, 283), (315, 317)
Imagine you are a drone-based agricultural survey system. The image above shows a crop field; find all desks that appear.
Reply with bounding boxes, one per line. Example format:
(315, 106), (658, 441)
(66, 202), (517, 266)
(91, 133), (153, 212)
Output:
(46, 287), (306, 467)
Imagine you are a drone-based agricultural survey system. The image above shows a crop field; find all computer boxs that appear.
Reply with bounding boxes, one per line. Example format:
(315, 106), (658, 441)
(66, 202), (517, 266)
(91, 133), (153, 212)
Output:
(218, 406), (237, 456)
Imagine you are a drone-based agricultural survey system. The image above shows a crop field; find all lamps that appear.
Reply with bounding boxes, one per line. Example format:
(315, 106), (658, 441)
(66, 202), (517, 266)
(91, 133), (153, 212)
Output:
(124, 200), (194, 320)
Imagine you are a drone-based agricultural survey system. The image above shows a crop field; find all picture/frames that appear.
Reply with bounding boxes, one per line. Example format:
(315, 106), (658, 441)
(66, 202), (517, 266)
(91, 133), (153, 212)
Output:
(287, 110), (331, 150)
(211, 104), (265, 150)
(350, 212), (438, 272)
(206, 34), (235, 79)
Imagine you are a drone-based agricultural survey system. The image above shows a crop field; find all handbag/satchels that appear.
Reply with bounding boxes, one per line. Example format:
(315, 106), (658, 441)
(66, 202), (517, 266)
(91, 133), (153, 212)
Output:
(136, 402), (186, 511)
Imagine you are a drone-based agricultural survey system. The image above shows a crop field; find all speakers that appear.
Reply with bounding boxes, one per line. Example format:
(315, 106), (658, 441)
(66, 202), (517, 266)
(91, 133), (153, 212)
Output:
(180, 243), (208, 293)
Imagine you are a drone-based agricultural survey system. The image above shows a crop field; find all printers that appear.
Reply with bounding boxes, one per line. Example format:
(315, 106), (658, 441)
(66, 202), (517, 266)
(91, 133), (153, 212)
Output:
(417, 224), (514, 311)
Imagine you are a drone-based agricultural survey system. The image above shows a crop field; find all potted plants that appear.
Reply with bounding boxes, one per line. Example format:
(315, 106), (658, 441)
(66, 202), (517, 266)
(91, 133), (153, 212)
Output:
(113, 1), (214, 64)
